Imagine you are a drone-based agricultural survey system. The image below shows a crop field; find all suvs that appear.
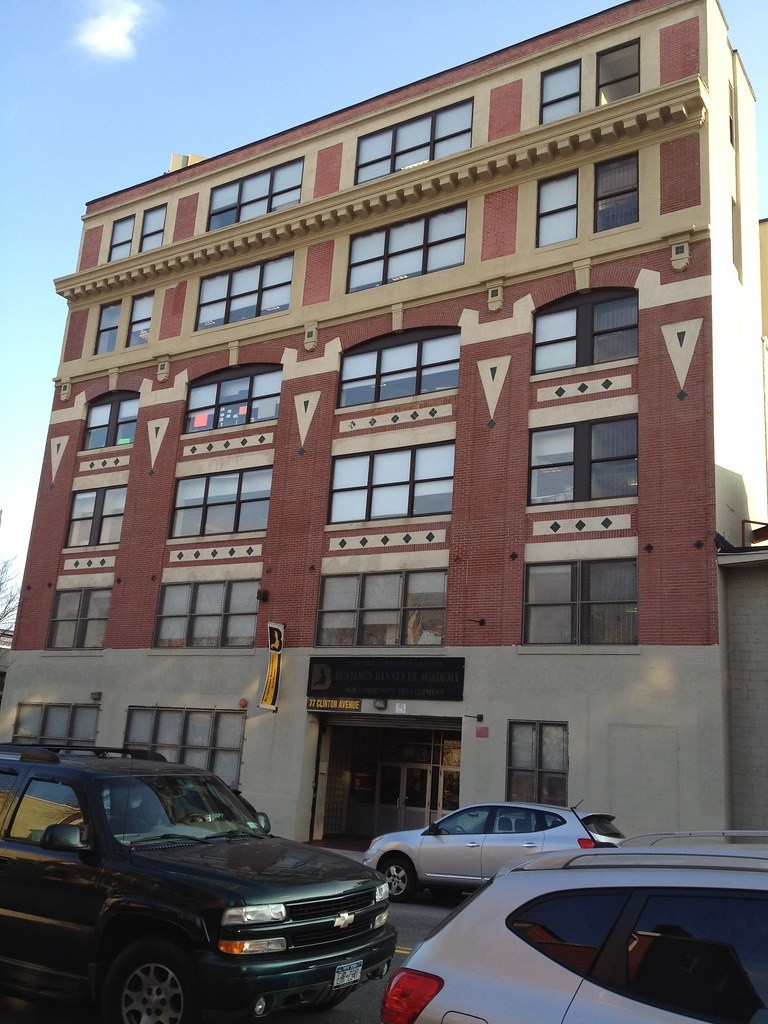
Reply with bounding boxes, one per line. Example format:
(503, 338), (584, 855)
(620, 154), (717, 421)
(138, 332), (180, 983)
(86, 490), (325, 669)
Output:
(379, 823), (768, 1024)
(359, 802), (627, 904)
(0, 742), (400, 1024)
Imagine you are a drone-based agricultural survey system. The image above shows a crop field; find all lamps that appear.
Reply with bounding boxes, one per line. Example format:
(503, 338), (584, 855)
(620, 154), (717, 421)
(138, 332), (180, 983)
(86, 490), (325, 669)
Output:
(373, 698), (387, 709)
(91, 692), (102, 700)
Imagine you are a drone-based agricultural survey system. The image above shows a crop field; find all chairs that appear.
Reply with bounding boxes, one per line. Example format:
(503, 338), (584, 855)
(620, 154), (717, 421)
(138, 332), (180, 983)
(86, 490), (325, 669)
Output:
(498, 817), (512, 833)
(514, 818), (532, 832)
(110, 788), (150, 834)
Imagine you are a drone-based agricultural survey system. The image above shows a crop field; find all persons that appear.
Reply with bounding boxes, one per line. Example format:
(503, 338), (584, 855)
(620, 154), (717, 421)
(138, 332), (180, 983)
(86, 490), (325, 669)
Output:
(126, 784), (211, 833)
